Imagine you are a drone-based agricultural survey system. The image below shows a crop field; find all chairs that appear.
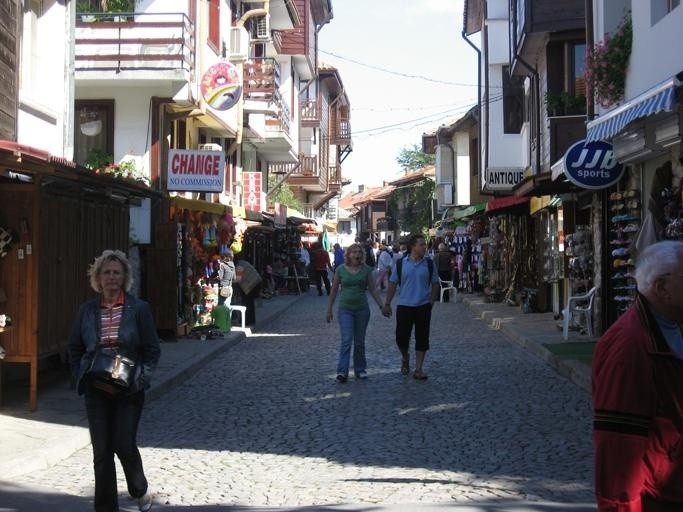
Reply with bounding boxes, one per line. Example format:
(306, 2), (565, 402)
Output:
(562, 286), (596, 340)
(439, 276), (457, 304)
(224, 286), (247, 328)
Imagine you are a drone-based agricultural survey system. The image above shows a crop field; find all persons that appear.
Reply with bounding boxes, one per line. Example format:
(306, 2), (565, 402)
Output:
(385, 235), (440, 380)
(593, 240), (683, 512)
(232, 259), (263, 325)
(66, 249), (161, 512)
(311, 235), (454, 304)
(326, 242), (390, 381)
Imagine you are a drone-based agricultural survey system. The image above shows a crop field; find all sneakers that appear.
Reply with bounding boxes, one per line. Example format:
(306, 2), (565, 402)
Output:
(336, 373), (346, 381)
(357, 371), (367, 379)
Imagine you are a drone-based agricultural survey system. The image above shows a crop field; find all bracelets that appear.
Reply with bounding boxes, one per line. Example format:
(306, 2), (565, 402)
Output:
(379, 304), (383, 309)
(385, 304), (390, 305)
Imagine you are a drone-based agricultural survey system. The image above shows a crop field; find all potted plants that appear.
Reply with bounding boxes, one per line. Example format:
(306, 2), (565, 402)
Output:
(545, 89), (586, 116)
(107, 1), (128, 22)
(76, 0), (98, 23)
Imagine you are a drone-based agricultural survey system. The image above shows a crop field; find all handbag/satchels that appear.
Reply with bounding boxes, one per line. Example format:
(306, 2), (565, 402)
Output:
(82, 345), (137, 396)
(220, 286), (232, 298)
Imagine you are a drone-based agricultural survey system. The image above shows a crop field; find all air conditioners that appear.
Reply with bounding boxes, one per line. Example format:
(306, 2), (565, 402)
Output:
(231, 25), (251, 65)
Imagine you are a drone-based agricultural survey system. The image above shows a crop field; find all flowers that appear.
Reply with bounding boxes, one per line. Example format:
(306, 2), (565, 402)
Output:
(580, 7), (631, 111)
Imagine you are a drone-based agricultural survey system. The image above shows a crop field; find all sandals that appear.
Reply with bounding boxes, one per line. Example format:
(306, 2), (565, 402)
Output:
(138, 492), (154, 512)
(401, 359), (410, 375)
(413, 371), (428, 381)
